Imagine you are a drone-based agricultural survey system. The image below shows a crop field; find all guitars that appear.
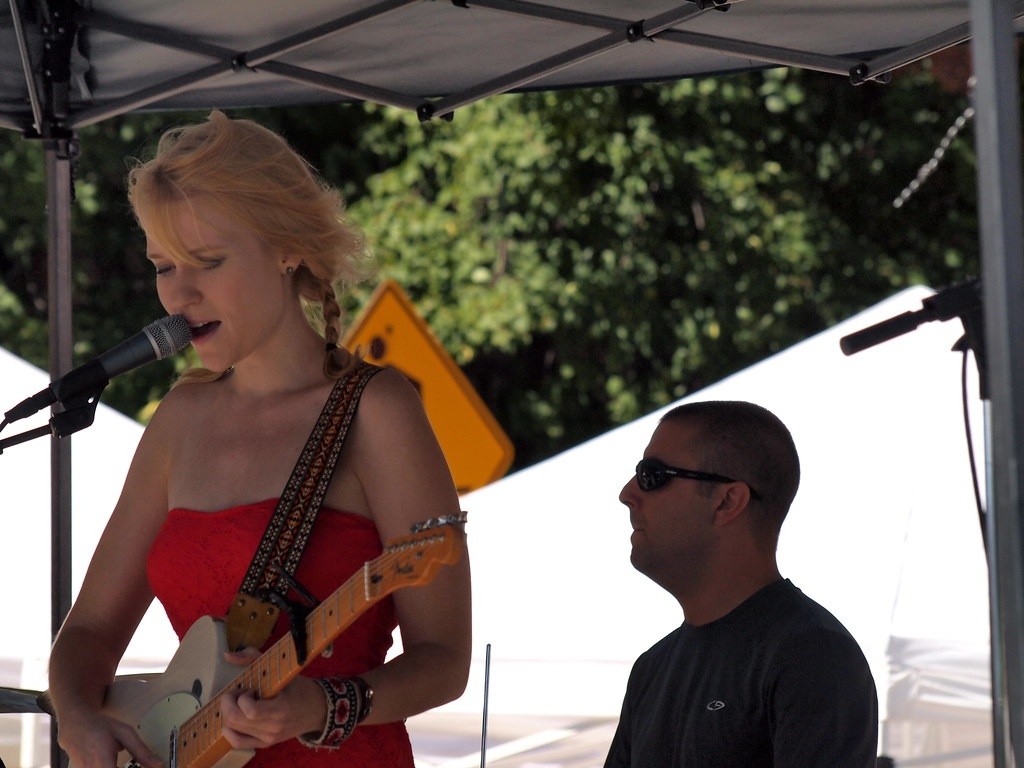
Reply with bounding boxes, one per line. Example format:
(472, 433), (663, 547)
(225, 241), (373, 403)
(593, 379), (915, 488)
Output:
(68, 510), (472, 768)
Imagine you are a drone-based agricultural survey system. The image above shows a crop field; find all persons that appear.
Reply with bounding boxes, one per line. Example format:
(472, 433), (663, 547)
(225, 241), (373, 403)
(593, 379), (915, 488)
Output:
(603, 400), (879, 768)
(47, 108), (472, 768)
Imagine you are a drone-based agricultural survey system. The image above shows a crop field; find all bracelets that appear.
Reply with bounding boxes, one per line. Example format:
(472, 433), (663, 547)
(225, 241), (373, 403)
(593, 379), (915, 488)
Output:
(295, 678), (360, 749)
(351, 674), (373, 722)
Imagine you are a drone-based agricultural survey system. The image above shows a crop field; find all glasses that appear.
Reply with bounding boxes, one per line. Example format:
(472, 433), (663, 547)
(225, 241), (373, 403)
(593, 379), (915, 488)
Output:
(636, 458), (764, 502)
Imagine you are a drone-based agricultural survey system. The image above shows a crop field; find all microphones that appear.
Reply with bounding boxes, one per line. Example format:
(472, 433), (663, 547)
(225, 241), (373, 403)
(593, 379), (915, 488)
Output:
(4, 314), (196, 424)
